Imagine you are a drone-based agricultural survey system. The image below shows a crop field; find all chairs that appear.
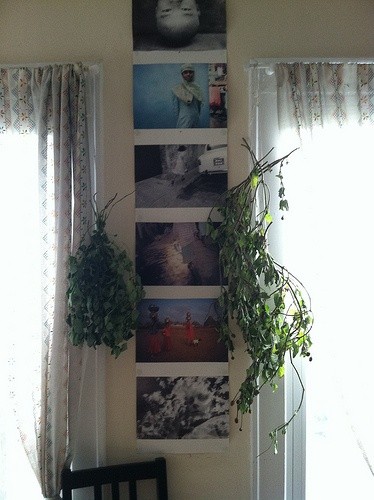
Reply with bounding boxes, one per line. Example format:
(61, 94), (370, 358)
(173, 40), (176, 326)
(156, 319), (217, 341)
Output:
(59, 456), (171, 500)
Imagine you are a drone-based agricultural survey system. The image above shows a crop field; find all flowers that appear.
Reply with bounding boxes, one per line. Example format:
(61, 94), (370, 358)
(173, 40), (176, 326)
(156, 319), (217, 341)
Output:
(64, 192), (148, 359)
(194, 133), (320, 463)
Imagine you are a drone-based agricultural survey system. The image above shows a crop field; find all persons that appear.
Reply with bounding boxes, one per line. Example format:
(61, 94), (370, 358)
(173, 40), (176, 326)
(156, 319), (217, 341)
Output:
(170, 64), (202, 128)
(156, 1), (201, 47)
(179, 385), (229, 439)
